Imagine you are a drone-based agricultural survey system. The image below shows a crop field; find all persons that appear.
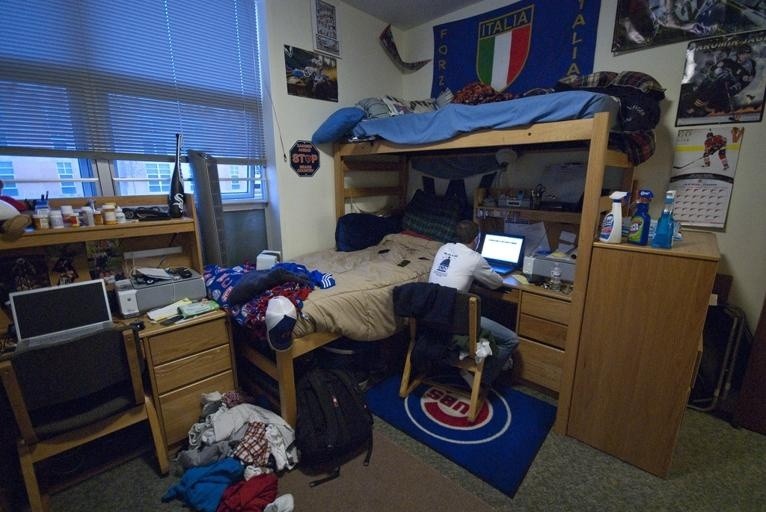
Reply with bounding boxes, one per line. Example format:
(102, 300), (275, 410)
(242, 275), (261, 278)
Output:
(678, 40), (759, 116)
(428, 220), (519, 403)
(701, 131), (731, 171)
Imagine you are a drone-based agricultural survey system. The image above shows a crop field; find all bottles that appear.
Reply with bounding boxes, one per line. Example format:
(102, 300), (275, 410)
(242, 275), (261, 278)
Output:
(32, 204), (126, 229)
(552, 272), (561, 292)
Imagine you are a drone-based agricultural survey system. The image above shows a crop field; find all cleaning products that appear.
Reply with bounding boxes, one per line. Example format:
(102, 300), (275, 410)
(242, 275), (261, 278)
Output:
(652, 188), (677, 249)
(596, 191), (632, 244)
(628, 190), (653, 244)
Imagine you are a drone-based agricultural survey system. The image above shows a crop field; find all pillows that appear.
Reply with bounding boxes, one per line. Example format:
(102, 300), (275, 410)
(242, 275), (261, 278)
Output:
(557, 71), (664, 101)
(400, 190), (466, 243)
(311, 96), (438, 143)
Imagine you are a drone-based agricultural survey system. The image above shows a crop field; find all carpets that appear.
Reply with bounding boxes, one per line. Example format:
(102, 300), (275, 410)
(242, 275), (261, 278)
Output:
(360, 367), (557, 497)
(275, 429), (498, 512)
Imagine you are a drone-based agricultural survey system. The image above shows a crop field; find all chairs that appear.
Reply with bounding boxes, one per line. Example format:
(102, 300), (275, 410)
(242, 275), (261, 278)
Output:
(400, 290), (501, 421)
(0, 330), (170, 512)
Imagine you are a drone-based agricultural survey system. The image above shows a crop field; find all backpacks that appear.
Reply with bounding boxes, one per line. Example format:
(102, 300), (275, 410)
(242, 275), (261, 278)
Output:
(292, 355), (375, 476)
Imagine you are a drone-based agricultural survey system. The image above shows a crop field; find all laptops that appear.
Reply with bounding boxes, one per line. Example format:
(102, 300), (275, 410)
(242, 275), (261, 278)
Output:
(8, 279), (113, 355)
(479, 231), (526, 275)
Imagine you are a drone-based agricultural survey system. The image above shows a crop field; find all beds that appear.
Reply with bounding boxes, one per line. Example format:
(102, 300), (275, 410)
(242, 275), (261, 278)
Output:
(201, 234), (447, 423)
(333, 112), (641, 430)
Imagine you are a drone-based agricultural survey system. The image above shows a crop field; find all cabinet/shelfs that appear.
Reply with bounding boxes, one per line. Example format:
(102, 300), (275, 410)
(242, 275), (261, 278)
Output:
(470, 189), (581, 398)
(567, 232), (720, 480)
(0, 193), (238, 444)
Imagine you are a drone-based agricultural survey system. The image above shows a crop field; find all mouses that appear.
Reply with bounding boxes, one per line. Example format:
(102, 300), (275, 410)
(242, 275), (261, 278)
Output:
(131, 321), (145, 330)
